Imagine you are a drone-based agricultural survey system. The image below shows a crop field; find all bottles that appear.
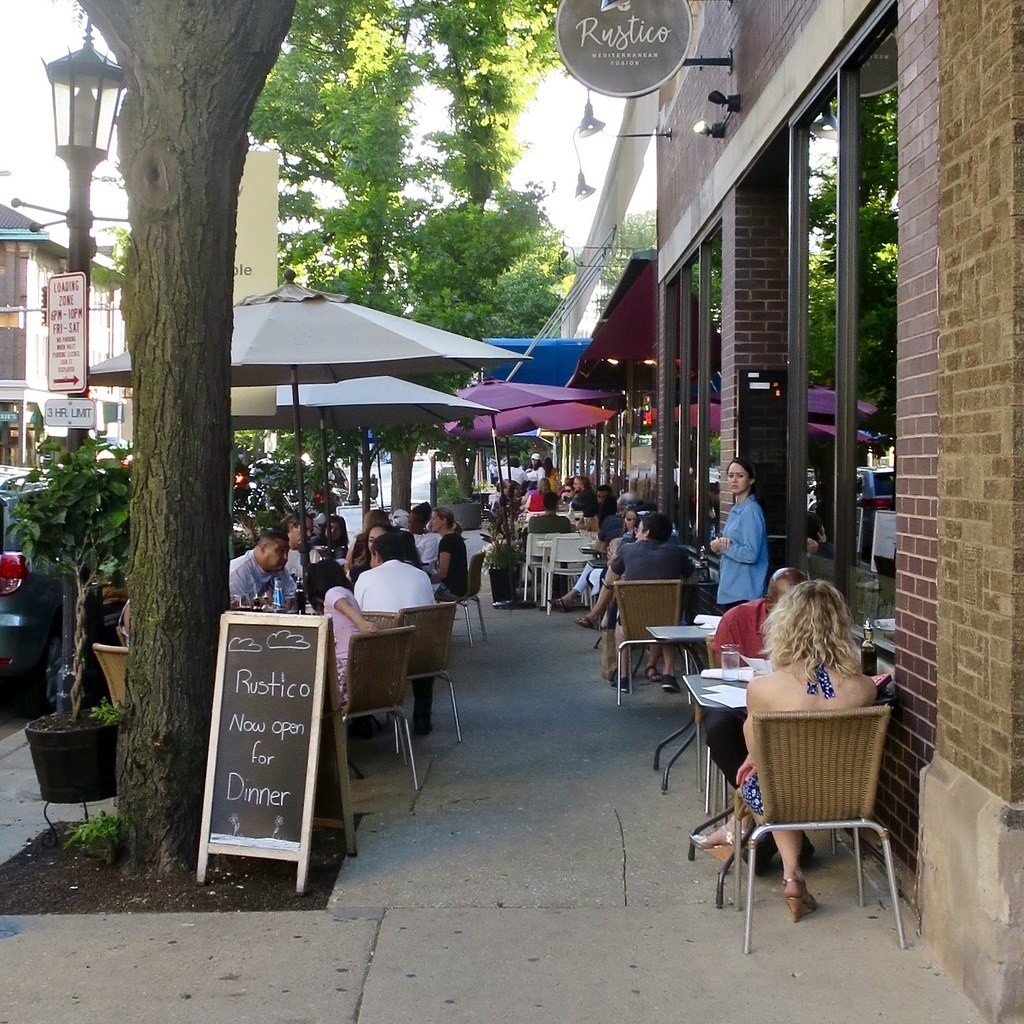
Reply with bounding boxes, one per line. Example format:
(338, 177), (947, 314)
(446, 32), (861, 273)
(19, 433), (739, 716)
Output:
(699, 548), (710, 579)
(272, 577), (284, 608)
(860, 627), (877, 676)
(295, 584), (306, 615)
(720, 644), (740, 683)
(231, 592), (272, 613)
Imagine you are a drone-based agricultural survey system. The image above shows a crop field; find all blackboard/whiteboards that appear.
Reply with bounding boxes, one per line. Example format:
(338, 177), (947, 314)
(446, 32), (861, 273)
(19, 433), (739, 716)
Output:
(336, 504), (379, 548)
(199, 611), (353, 861)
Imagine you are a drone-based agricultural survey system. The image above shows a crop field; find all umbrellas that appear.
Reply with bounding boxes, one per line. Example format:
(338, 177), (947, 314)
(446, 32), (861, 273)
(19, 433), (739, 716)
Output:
(91, 269), (619, 613)
(651, 373), (878, 443)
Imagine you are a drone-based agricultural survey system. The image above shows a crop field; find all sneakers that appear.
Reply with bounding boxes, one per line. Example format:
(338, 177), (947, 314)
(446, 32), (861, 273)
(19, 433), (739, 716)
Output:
(611, 677), (628, 692)
(661, 675), (679, 691)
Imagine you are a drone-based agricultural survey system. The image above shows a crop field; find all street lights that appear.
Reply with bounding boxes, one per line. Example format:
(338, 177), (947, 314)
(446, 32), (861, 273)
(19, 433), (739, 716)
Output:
(40, 16), (127, 453)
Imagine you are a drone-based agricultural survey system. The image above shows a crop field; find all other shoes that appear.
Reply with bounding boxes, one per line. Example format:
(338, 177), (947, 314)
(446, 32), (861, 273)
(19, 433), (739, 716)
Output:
(547, 597), (570, 612)
(741, 845), (771, 875)
(414, 716), (432, 734)
(780, 830), (816, 868)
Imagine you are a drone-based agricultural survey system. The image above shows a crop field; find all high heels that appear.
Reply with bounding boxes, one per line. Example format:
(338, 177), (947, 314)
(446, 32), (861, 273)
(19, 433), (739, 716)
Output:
(689, 826), (739, 867)
(783, 876), (816, 923)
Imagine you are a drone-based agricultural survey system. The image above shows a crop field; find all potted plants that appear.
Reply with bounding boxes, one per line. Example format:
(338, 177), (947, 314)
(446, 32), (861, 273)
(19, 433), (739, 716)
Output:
(482, 545), (516, 603)
(436, 466), (481, 530)
(0, 431), (131, 803)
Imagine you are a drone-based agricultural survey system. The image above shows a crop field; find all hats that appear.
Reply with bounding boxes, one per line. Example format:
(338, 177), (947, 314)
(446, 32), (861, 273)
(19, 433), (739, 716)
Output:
(530, 453), (540, 460)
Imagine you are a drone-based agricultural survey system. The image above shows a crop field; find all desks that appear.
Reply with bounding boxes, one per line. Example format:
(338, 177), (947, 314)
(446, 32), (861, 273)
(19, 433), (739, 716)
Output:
(682, 675), (898, 909)
(647, 626), (715, 795)
(526, 512), (583, 518)
(578, 547), (603, 559)
(587, 559), (608, 568)
(602, 577), (718, 677)
(536, 541), (589, 609)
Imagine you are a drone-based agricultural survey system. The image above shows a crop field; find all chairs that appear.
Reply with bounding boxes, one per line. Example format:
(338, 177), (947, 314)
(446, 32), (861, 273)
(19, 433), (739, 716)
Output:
(523, 534), (907, 954)
(93, 643), (128, 706)
(434, 549), (486, 648)
(344, 601), (461, 791)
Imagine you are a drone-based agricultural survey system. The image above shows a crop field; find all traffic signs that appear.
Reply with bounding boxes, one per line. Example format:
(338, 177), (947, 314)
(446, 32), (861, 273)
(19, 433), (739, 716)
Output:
(45, 272), (87, 393)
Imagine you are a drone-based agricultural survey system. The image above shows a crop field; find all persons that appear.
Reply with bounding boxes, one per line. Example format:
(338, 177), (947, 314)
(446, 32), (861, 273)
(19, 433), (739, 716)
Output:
(704, 567), (816, 874)
(229, 501), (469, 733)
(687, 579), (877, 923)
(486, 453), (699, 693)
(710, 459), (769, 612)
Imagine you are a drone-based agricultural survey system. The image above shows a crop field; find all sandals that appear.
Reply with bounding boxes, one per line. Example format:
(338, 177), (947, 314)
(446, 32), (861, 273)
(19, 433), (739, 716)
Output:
(644, 664), (662, 682)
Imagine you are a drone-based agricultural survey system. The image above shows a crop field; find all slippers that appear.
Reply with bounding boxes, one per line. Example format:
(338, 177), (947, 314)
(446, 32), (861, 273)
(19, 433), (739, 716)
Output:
(574, 612), (603, 630)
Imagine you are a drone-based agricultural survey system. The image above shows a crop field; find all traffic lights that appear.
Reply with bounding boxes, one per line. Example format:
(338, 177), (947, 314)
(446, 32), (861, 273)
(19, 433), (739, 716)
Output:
(234, 442), (247, 488)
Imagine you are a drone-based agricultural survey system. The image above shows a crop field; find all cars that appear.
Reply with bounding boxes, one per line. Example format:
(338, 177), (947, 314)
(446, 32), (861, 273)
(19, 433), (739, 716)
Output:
(246, 456), (359, 523)
(575, 451), (720, 504)
(381, 446), (423, 464)
(0, 436), (138, 719)
(807, 465), (897, 574)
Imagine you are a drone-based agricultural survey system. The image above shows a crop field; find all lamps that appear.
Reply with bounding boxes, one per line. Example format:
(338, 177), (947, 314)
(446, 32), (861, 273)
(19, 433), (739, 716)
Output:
(578, 89), (606, 138)
(693, 89), (740, 138)
(573, 126), (672, 201)
